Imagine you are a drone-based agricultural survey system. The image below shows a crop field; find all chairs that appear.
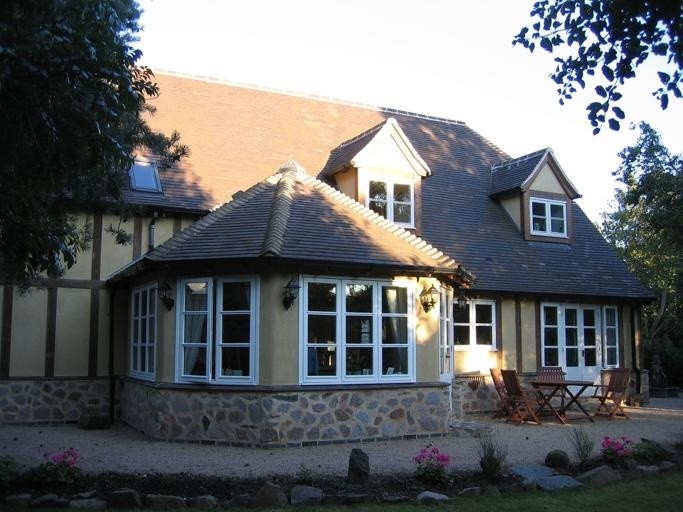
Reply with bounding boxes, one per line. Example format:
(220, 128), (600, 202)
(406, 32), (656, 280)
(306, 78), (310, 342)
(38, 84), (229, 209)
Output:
(490, 366), (632, 425)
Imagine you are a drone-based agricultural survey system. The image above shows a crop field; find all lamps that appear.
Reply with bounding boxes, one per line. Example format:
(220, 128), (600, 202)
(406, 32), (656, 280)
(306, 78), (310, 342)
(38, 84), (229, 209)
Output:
(423, 284), (440, 312)
(157, 281), (174, 311)
(283, 280), (300, 310)
